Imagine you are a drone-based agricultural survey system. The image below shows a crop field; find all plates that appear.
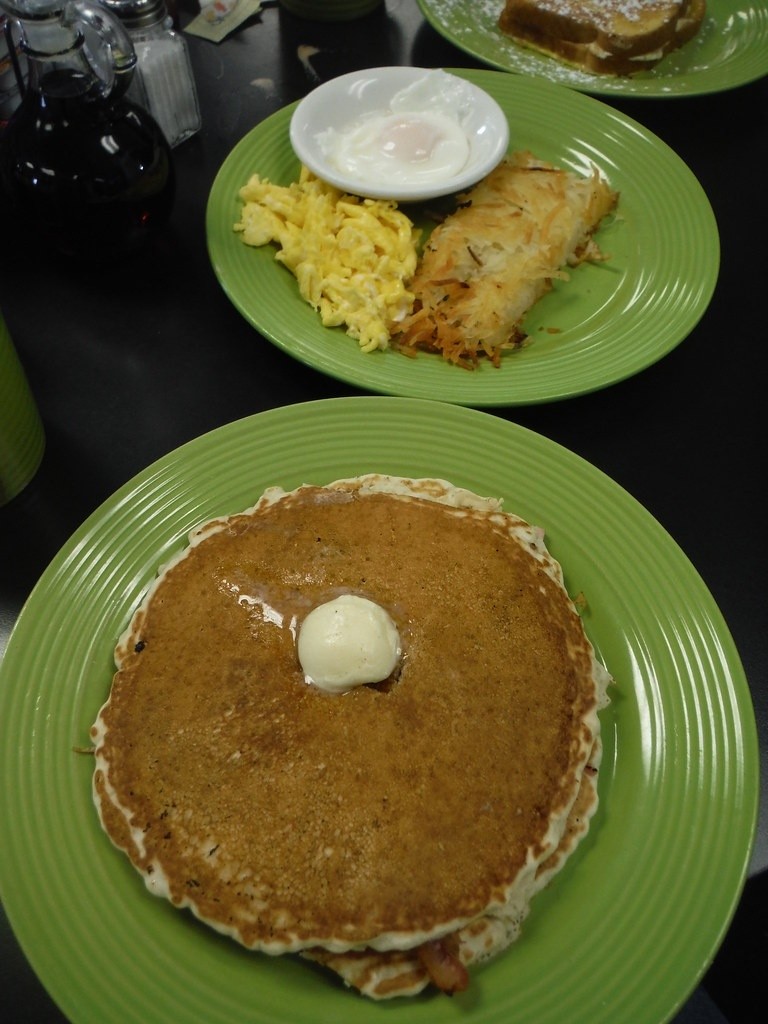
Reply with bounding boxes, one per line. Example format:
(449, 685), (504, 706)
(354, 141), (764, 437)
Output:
(1, 397), (760, 1024)
(289, 66), (509, 204)
(204, 70), (721, 408)
(416, 0), (768, 100)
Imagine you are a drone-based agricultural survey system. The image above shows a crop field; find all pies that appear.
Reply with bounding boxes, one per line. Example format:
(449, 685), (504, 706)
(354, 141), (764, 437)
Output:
(89, 473), (613, 1003)
(388, 154), (621, 369)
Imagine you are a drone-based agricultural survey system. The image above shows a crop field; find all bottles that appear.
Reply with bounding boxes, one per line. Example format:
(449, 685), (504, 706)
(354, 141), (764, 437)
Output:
(92, 1), (202, 150)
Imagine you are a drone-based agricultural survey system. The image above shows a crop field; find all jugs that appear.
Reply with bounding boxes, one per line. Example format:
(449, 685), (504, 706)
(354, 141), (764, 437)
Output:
(0, 1), (175, 256)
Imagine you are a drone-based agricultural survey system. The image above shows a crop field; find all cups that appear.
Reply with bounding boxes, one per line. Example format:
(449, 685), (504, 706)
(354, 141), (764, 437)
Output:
(1, 311), (47, 508)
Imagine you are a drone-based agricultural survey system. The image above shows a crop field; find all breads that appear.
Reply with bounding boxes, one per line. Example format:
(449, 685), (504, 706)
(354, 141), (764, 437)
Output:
(498, 0), (707, 77)
